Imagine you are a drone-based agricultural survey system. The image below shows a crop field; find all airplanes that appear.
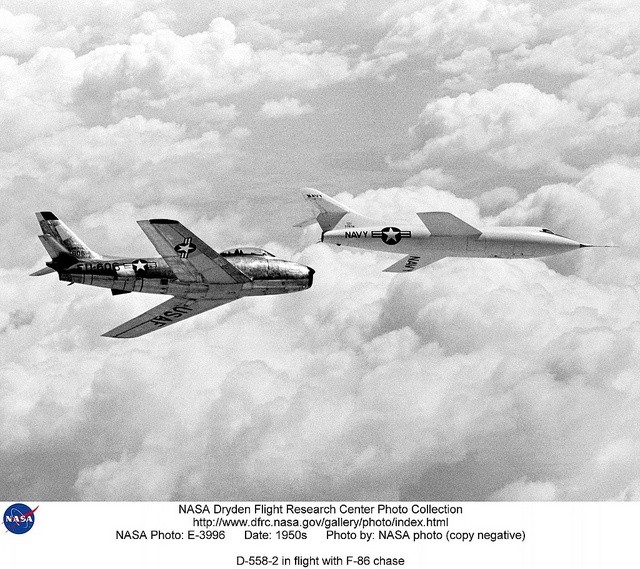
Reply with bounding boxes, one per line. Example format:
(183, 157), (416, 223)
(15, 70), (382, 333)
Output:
(294, 188), (620, 272)
(29, 211), (315, 338)
